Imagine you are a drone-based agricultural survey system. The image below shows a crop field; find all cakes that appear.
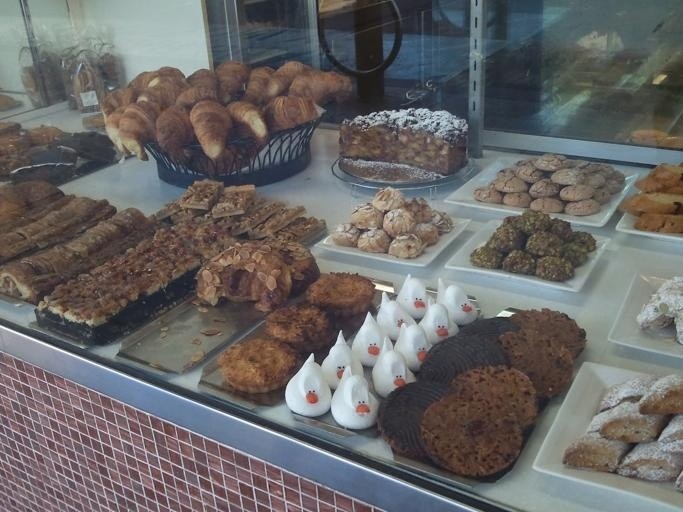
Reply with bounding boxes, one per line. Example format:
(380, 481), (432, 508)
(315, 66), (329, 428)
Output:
(336, 107), (469, 183)
(285, 275), (478, 429)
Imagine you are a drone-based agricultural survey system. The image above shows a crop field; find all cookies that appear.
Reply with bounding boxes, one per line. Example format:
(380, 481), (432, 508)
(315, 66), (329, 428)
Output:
(151, 178), (329, 250)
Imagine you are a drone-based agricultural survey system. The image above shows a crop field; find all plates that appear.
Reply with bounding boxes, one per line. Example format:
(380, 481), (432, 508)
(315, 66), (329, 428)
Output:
(614, 191), (682, 248)
(441, 218), (612, 294)
(330, 158), (474, 190)
(604, 269), (682, 359)
(441, 153), (640, 228)
(313, 216), (470, 267)
(529, 360), (682, 508)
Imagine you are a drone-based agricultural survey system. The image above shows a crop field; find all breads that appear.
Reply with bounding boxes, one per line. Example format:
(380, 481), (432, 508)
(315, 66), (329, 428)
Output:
(471, 205), (599, 284)
(561, 371), (680, 494)
(217, 270), (377, 392)
(620, 159), (683, 237)
(474, 152), (626, 217)
(372, 309), (587, 480)
(1, 180), (147, 309)
(636, 273), (683, 346)
(32, 225), (325, 345)
(334, 185), (453, 260)
(96, 56), (348, 161)
(1, 116), (110, 192)
